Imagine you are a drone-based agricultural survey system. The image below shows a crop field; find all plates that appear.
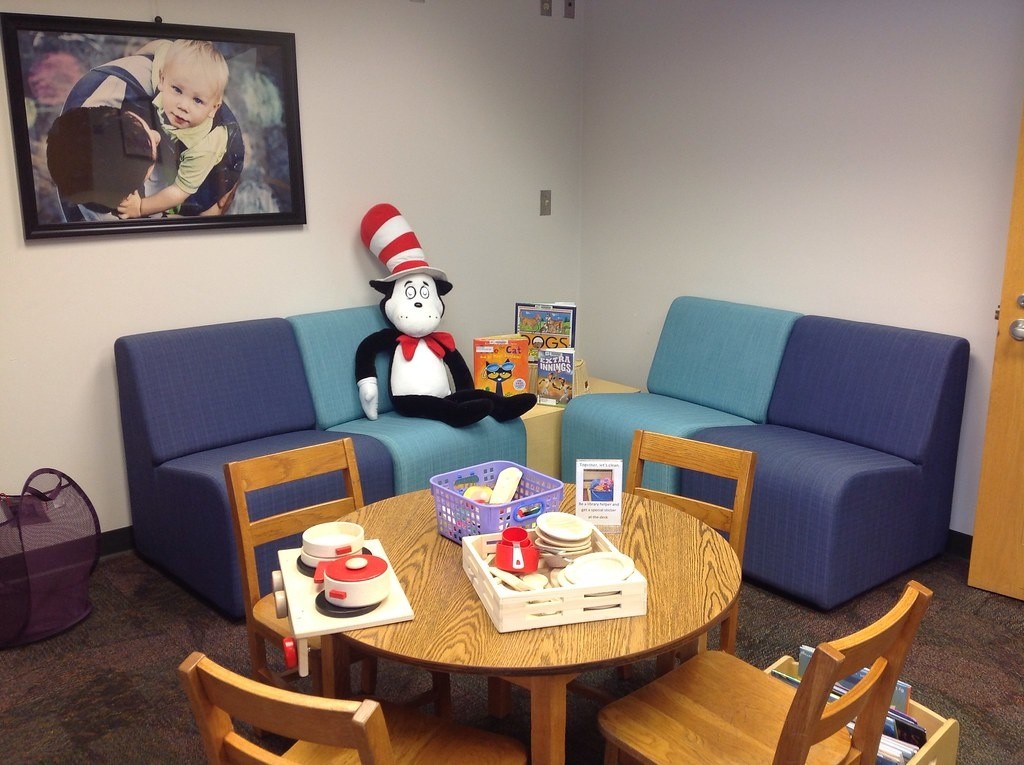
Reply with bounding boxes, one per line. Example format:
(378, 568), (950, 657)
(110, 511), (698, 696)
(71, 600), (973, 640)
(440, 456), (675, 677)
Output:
(535, 512), (593, 551)
(559, 553), (634, 588)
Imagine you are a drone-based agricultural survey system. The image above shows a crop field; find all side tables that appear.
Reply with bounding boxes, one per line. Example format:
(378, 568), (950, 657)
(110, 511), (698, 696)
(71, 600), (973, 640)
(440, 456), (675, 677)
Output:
(508, 371), (642, 483)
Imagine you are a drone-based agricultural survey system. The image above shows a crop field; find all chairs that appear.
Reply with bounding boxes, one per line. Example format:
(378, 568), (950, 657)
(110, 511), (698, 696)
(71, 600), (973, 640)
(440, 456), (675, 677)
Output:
(597, 580), (935, 765)
(178, 651), (528, 765)
(225, 437), (378, 694)
(617, 428), (757, 680)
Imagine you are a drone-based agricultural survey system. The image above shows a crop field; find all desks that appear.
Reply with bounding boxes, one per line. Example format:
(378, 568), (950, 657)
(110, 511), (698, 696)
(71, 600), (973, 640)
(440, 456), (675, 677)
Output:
(321, 480), (744, 765)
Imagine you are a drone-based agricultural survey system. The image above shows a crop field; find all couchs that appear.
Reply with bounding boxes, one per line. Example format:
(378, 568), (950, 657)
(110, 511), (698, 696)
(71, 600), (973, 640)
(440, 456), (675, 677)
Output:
(562, 295), (802, 497)
(116, 317), (395, 622)
(288, 303), (527, 495)
(681, 314), (970, 612)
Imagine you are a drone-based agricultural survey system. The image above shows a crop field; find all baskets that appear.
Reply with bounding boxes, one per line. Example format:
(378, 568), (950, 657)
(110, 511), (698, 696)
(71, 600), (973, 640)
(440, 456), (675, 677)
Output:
(428, 460), (563, 547)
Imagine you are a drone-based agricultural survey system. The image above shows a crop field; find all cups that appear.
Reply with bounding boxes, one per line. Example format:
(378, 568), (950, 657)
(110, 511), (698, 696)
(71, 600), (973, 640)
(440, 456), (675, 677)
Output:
(496, 542), (539, 572)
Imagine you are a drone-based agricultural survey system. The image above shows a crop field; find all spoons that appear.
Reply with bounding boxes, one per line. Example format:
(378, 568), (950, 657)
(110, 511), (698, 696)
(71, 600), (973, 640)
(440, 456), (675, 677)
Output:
(523, 574), (548, 603)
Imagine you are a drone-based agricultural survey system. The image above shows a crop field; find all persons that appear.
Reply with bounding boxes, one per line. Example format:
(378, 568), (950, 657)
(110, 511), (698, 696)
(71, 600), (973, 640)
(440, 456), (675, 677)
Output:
(47, 40), (245, 224)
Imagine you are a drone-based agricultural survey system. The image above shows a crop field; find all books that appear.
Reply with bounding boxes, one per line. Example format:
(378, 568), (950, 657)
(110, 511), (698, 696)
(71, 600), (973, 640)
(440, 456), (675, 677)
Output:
(473, 302), (577, 408)
(770, 645), (927, 765)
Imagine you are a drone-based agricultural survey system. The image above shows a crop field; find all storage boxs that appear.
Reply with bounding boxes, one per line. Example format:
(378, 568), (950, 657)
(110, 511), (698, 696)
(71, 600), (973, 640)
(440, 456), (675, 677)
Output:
(763, 654), (959, 765)
(430, 459), (565, 545)
(459, 527), (649, 634)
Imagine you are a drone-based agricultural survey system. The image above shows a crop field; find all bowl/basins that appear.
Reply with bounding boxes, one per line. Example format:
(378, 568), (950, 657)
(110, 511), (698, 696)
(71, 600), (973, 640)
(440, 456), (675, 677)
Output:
(300, 521), (365, 568)
(324, 556), (390, 608)
(539, 547), (593, 567)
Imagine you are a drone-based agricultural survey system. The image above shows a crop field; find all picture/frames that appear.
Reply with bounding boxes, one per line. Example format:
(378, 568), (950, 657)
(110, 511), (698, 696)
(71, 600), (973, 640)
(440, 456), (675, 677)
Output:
(0, 12), (308, 239)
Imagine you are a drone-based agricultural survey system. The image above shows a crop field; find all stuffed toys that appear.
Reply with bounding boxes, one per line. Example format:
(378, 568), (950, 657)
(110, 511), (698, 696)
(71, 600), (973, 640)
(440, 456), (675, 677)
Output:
(354, 204), (537, 428)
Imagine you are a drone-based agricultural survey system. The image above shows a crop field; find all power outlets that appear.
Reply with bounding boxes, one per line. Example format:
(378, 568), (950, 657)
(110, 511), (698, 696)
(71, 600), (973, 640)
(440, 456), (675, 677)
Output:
(541, 0), (552, 16)
